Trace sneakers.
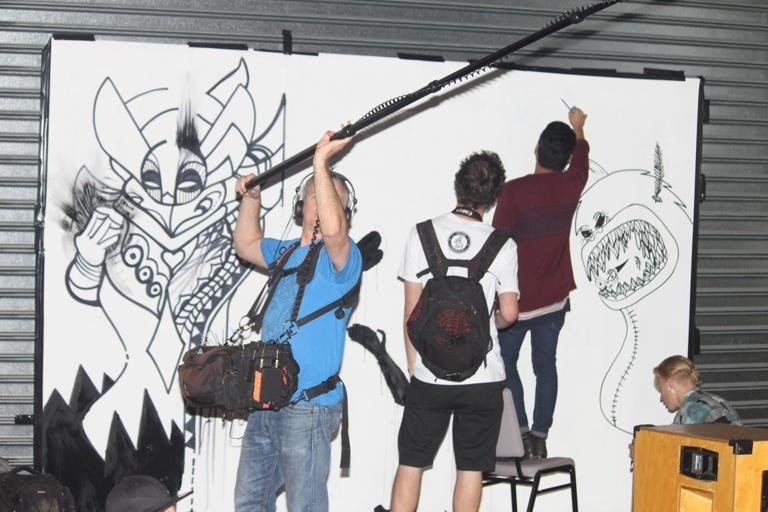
[519,432,547,461]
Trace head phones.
[292,171,357,229]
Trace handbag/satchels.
[178,340,300,421]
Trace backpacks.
[405,219,519,381]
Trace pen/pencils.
[562,99,573,111]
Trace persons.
[233,120,364,512]
[629,357,741,462]
[491,106,590,461]
[390,150,520,512]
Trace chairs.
[485,388,578,511]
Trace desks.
[633,423,767,511]
